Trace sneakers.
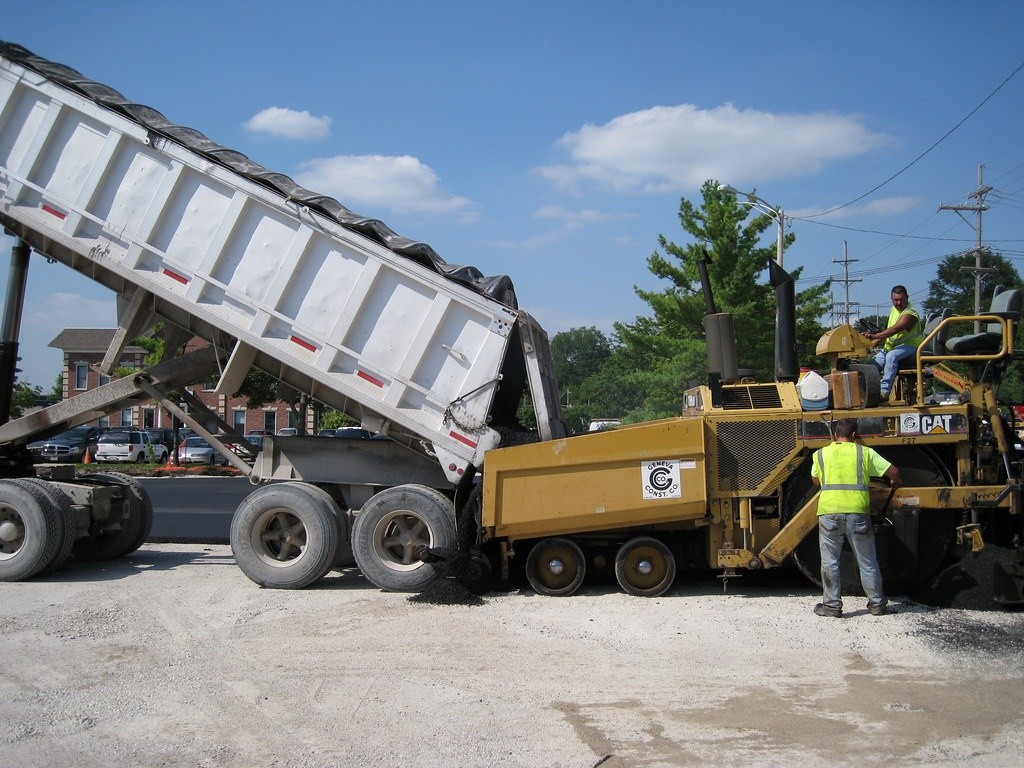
[867,602,886,615]
[814,603,842,618]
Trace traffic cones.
[83,446,92,464]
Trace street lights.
[716,184,784,382]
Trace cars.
[234,424,375,457]
[169,436,230,467]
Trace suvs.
[41,426,111,463]
[144,425,199,452]
[93,427,170,465]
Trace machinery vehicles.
[474,245,1023,609]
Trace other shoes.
[880,392,889,402]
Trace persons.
[861,285,923,403]
[810,418,902,618]
[798,360,820,382]
